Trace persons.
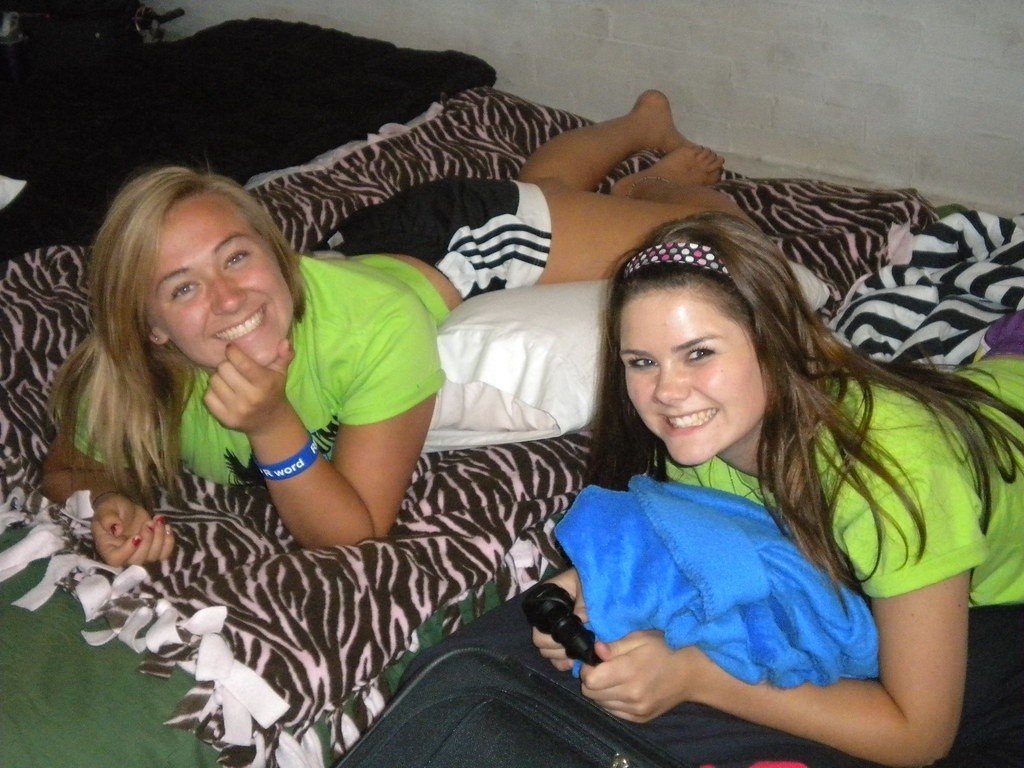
[39,85,749,569]
[529,209,1024,768]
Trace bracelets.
[251,430,320,481]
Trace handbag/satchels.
[332,647,691,768]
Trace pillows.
[420,258,832,451]
